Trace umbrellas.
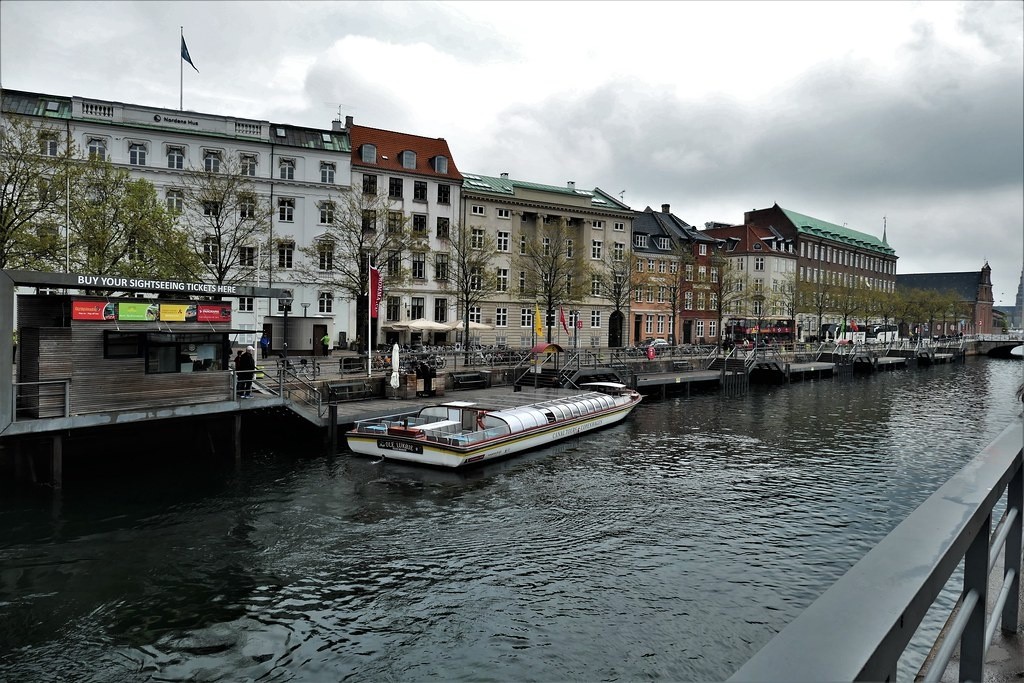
[389,342,400,398]
[381,317,495,346]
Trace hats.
[247,345,255,350]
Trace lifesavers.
[477,411,487,430]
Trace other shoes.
[240,395,246,398]
[246,394,254,398]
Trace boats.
[345,380,642,468]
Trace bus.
[820,323,899,344]
[724,317,796,351]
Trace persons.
[762,335,770,350]
[414,361,437,398]
[320,333,329,356]
[260,332,271,359]
[356,334,361,352]
[234,346,256,399]
[278,349,298,374]
[933,329,963,343]
[389,338,393,345]
[742,336,749,354]
[909,336,923,347]
[721,338,735,357]
[403,335,432,352]
[223,340,233,369]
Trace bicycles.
[372,350,448,372]
[282,360,315,382]
[471,347,523,366]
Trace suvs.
[623,338,669,356]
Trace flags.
[841,319,845,332]
[370,266,383,318]
[561,305,569,335]
[182,36,199,73]
[850,320,858,331]
[534,303,543,337]
